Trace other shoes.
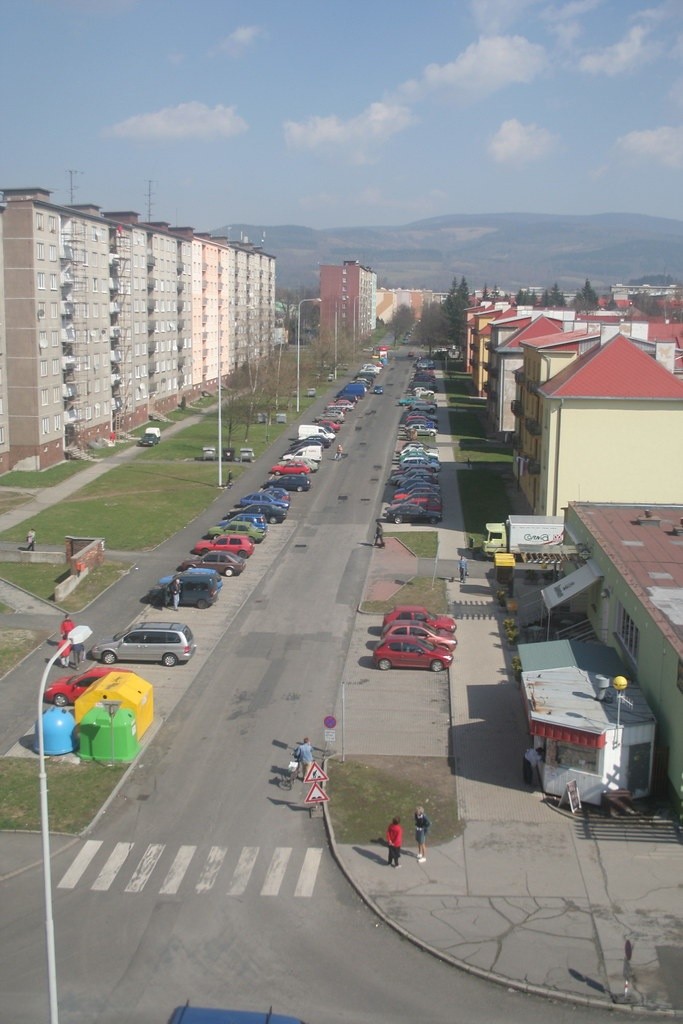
[61,664,64,668]
[64,666,68,668]
[76,666,79,670]
[417,854,422,858]
[395,864,401,870]
[418,858,426,862]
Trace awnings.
[540,561,605,641]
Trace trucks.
[297,424,336,443]
[283,445,322,463]
[482,515,564,562]
[342,384,364,390]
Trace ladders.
[116,413,120,440]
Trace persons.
[336,445,342,461]
[172,578,181,611]
[296,738,312,779]
[71,644,82,670]
[522,747,545,786]
[57,635,70,667]
[386,817,402,868]
[372,522,384,548]
[415,807,431,862]
[81,646,85,662]
[26,529,36,551]
[61,615,74,636]
[458,555,467,582]
[225,469,232,489]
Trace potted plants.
[511,656,522,682]
[503,618,520,645]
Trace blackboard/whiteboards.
[558,779,581,812]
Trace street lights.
[334,293,347,380]
[218,305,254,486]
[297,298,322,413]
[37,625,93,1024]
[353,294,367,363]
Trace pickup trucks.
[138,434,160,447]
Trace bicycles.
[289,754,300,790]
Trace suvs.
[264,475,312,492]
[148,568,223,609]
[216,514,268,532]
[91,621,196,667]
[194,534,255,559]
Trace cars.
[373,604,457,672]
[260,488,291,501]
[386,356,443,525]
[363,347,373,352]
[272,362,384,476]
[181,550,246,577]
[44,666,136,706]
[207,521,266,544]
[227,504,287,524]
[408,352,414,356]
[240,492,290,510]
[373,386,383,394]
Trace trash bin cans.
[203,447,216,461]
[240,448,253,462]
[277,414,287,424]
[306,388,316,396]
[258,413,268,423]
[223,447,235,461]
